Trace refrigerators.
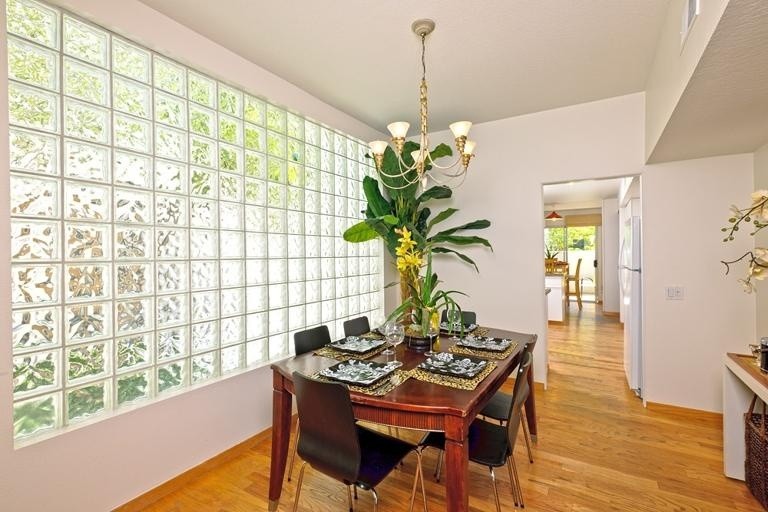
[617,196,642,402]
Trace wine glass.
[750,342,762,367]
[449,310,460,340]
[424,311,440,356]
[377,316,395,355]
[386,322,403,368]
[412,310,420,325]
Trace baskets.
[744,412,768,509]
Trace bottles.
[760,337,768,375]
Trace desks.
[722,353,768,481]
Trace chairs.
[343,315,370,337]
[408,345,532,509]
[442,310,477,327]
[286,324,331,482]
[290,369,429,512]
[566,258,582,311]
[478,334,535,466]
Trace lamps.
[545,204,563,220]
[368,17,477,190]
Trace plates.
[440,321,479,332]
[417,351,489,379]
[318,357,396,386]
[456,333,512,353]
[324,335,387,354]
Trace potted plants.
[545,244,559,268]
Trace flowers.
[721,188,768,294]
[382,226,473,343]
